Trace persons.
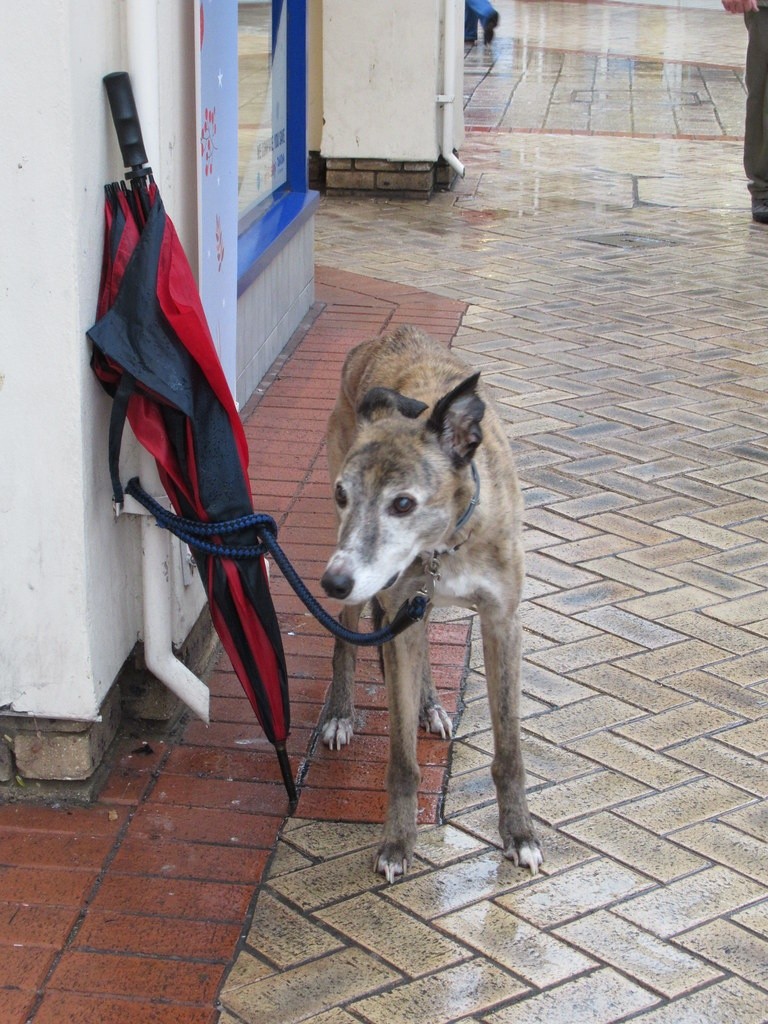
[465,0,501,45]
[721,0,768,221]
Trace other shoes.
[484,11,498,44]
[751,200,768,224]
[464,38,475,43]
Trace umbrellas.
[86,72,296,811]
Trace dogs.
[314,321,548,887]
